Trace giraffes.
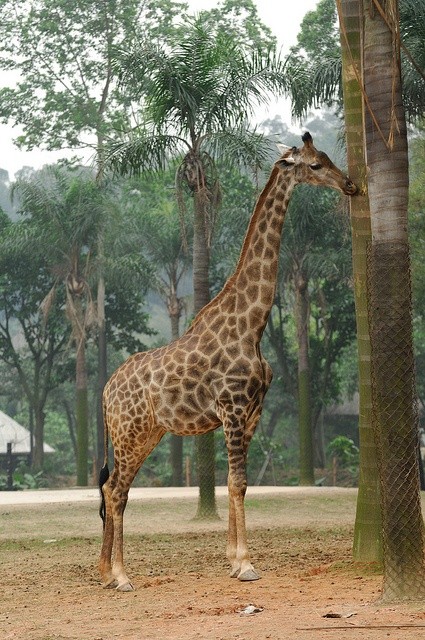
[98,130,358,592]
